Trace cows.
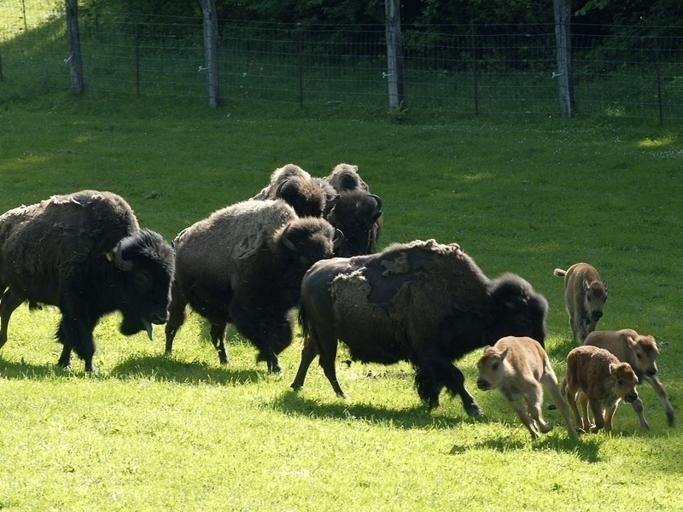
[549,260,611,341]
[0,185,179,375]
[251,160,388,266]
[166,195,346,377]
[475,334,584,450]
[289,236,552,422]
[585,325,679,437]
[554,342,646,434]
